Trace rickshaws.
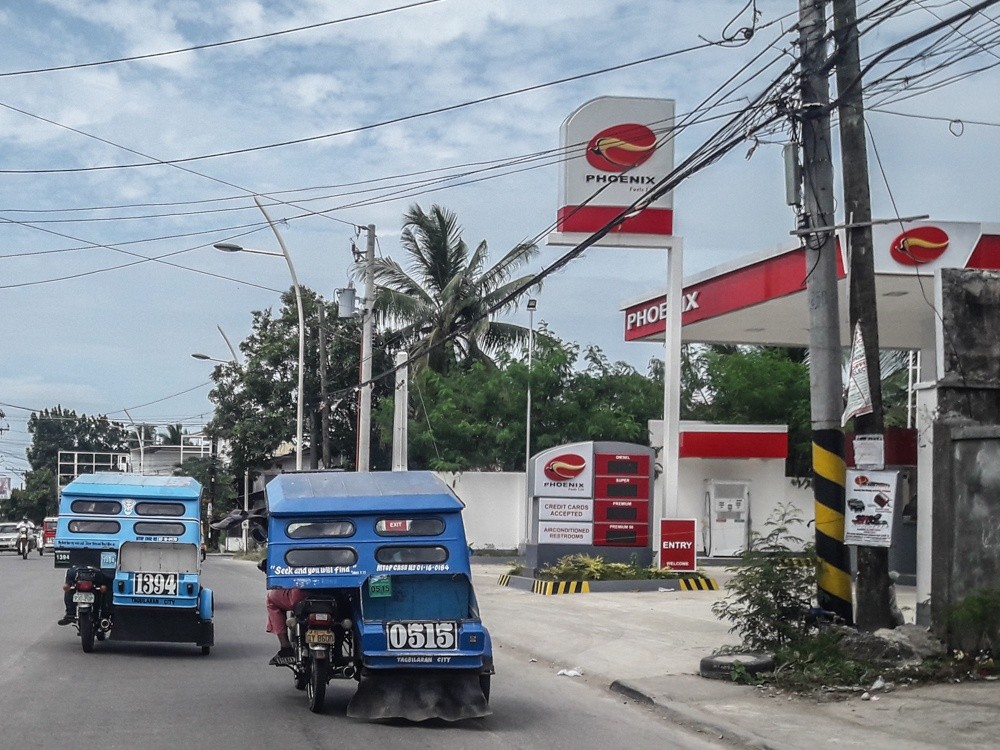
[251,467,496,724]
[53,471,216,656]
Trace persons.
[257,523,335,658]
[58,502,119,625]
[16,516,36,551]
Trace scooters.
[13,524,43,559]
[33,517,58,548]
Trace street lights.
[213,195,306,471]
[191,323,251,555]
[518,297,537,556]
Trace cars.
[0,522,21,550]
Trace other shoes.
[58,614,77,626]
[268,647,296,666]
